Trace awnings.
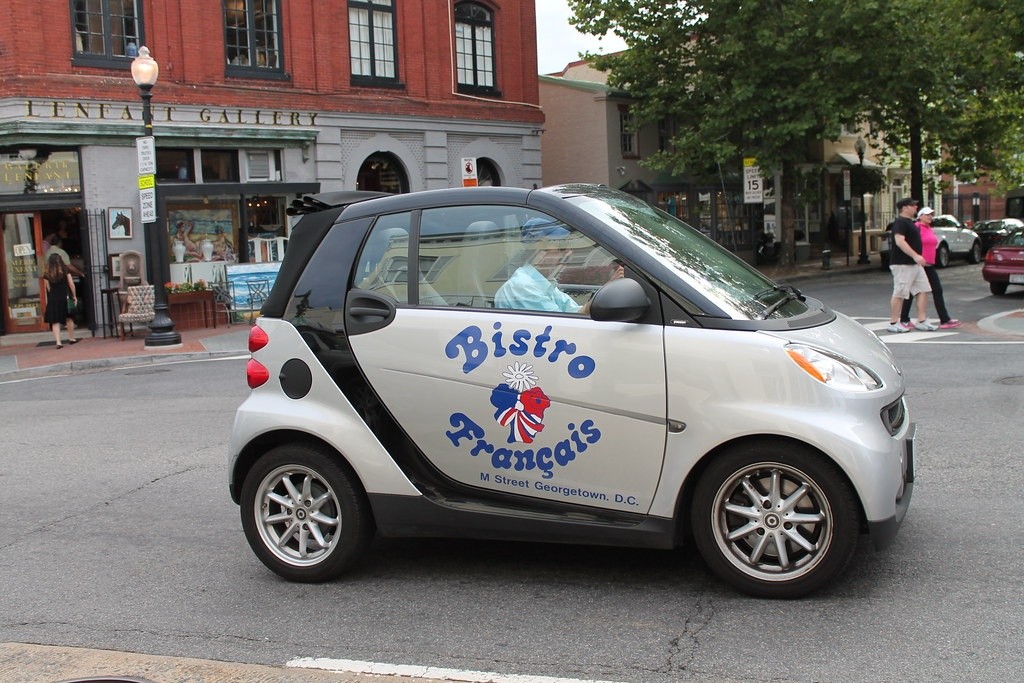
[836,153,886,170]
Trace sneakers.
[916,318,938,331]
[888,321,910,333]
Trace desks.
[167,290,217,329]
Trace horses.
[112,211,130,236]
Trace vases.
[172,240,185,263]
[201,239,213,262]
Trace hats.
[897,197,919,209]
[916,207,935,221]
[521,221,571,250]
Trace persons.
[494,216,624,315]
[42,227,56,253]
[44,235,85,328]
[900,207,962,331]
[44,253,79,349]
[888,198,939,331]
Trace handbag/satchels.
[67,299,82,313]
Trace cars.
[980,226,1024,296]
[224,182,918,600]
[970,215,1024,257]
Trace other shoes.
[57,344,64,349]
[940,318,961,329]
[70,338,77,344]
[901,319,916,329]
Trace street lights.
[852,136,872,265]
[129,45,184,346]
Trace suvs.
[877,213,984,272]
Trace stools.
[101,288,134,338]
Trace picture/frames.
[165,201,239,264]
[109,253,121,280]
[108,206,133,240]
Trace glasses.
[542,239,573,251]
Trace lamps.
[617,165,626,176]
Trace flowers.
[164,279,207,294]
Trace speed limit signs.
[741,156,764,205]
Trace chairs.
[245,280,271,325]
[119,285,155,342]
[368,227,447,308]
[248,236,289,263]
[211,281,237,327]
[457,220,511,309]
